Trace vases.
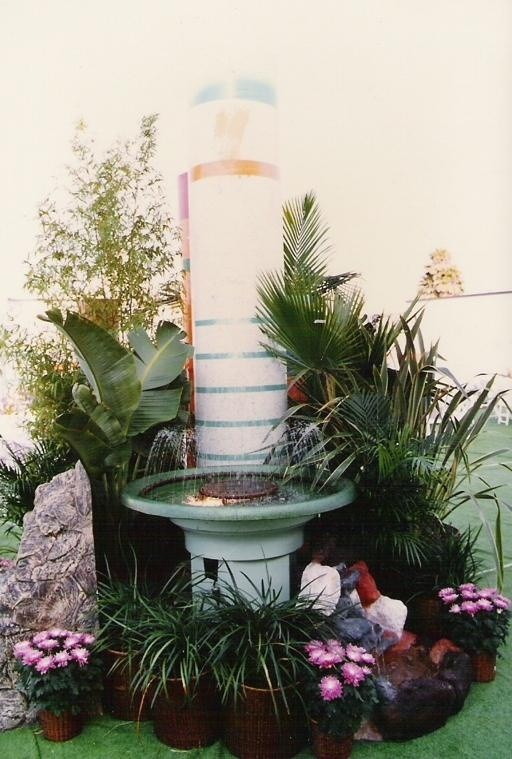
[463,655,499,684]
[39,705,80,740]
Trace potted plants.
[83,543,362,759]
[270,288,509,633]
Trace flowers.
[435,581,509,662]
[12,625,98,722]
[304,636,383,740]
